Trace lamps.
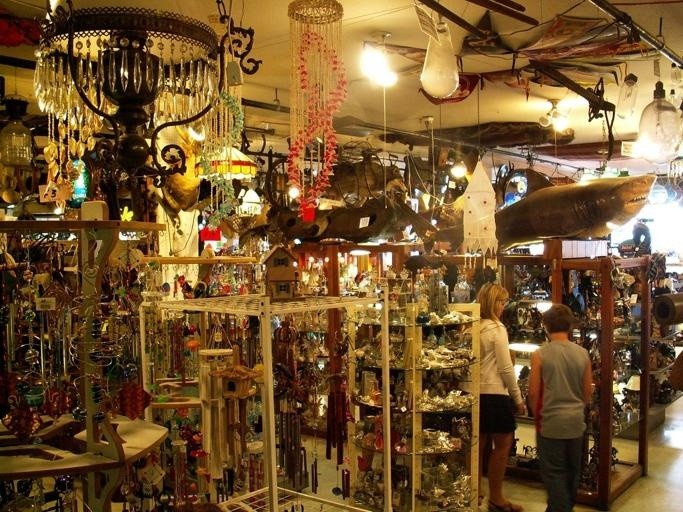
[33,0,224,225]
[195,143,258,185]
[450,142,467,181]
[634,41,682,161]
[535,92,565,129]
[0,61,33,170]
[237,185,262,220]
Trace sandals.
[487,499,523,512]
[477,495,484,506]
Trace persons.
[527,303,592,512]
[458,282,525,512]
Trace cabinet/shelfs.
[0,210,681,511]
